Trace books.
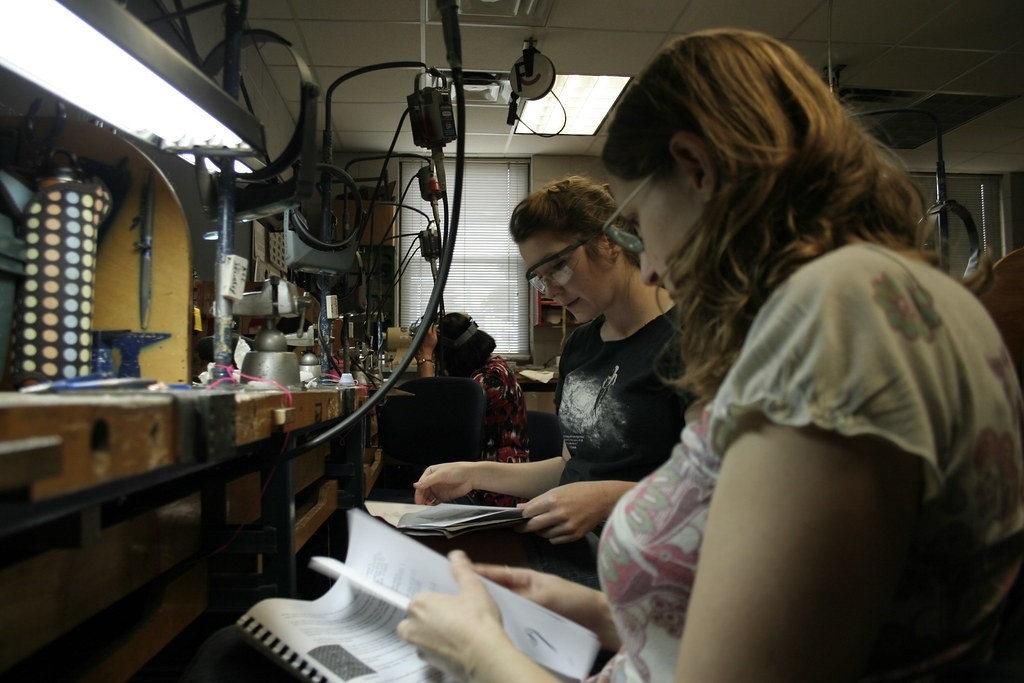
[236,501,600,683]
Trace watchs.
[417,359,435,364]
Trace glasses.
[603,166,661,252]
[525,235,595,295]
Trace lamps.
[0,0,267,174]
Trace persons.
[411,313,530,508]
[410,175,704,589]
[396,27,1023,683]
